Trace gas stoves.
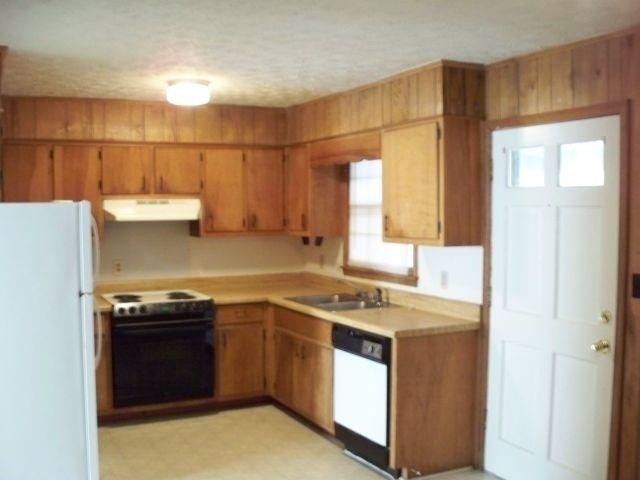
[99,287,215,319]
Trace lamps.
[166,78,210,106]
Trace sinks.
[317,302,400,313]
[283,293,360,305]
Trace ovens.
[108,311,220,414]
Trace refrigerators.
[1,199,103,480]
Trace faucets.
[354,288,381,305]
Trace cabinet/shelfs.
[190,146,287,237]
[284,142,349,238]
[212,302,267,409]
[102,142,203,194]
[381,115,484,247]
[0,140,104,242]
[266,303,336,436]
